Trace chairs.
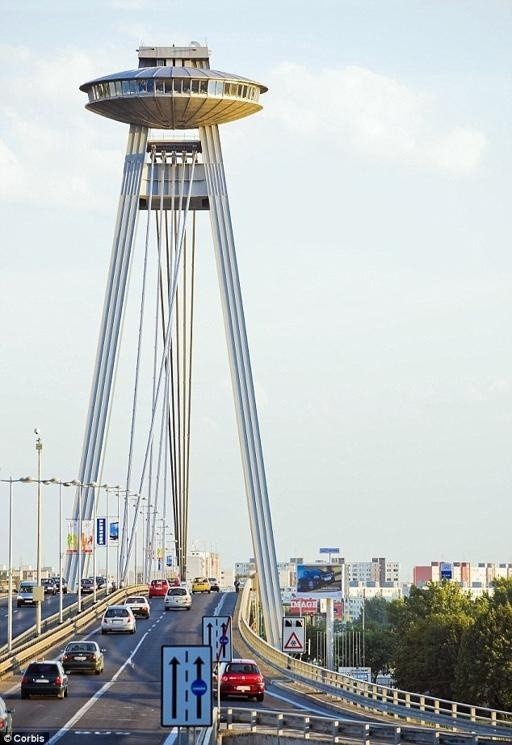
[244,666,250,673]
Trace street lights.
[0,475,187,651]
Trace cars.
[164,587,193,610]
[298,569,335,590]
[191,576,220,594]
[212,658,264,701]
[0,640,106,745]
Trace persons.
[234,579,241,593]
